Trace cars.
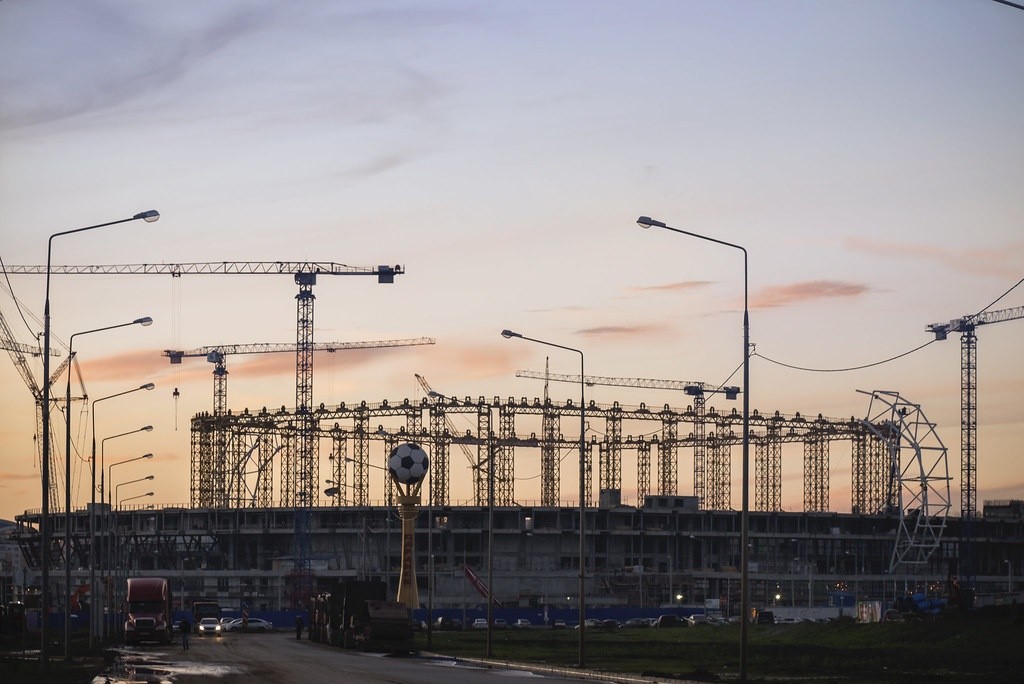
[512,619,532,630]
[198,618,223,638]
[494,618,508,631]
[554,618,567,629]
[577,610,829,627]
[219,616,273,632]
[472,618,488,630]
[415,616,462,632]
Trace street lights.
[501,328,588,667]
[326,479,366,585]
[638,214,750,683]
[41,208,159,678]
[66,316,153,666]
[428,391,493,658]
[108,453,153,653]
[114,475,155,640]
[100,426,154,646]
[91,383,157,660]
[345,456,393,602]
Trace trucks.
[192,599,222,631]
[121,574,175,647]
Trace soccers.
[388,444,429,483]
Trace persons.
[296,615,304,640]
[179,615,191,651]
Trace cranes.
[162,337,439,624]
[0,260,410,407]
[512,366,744,508]
[0,313,89,510]
[925,305,1024,521]
[414,371,481,509]
